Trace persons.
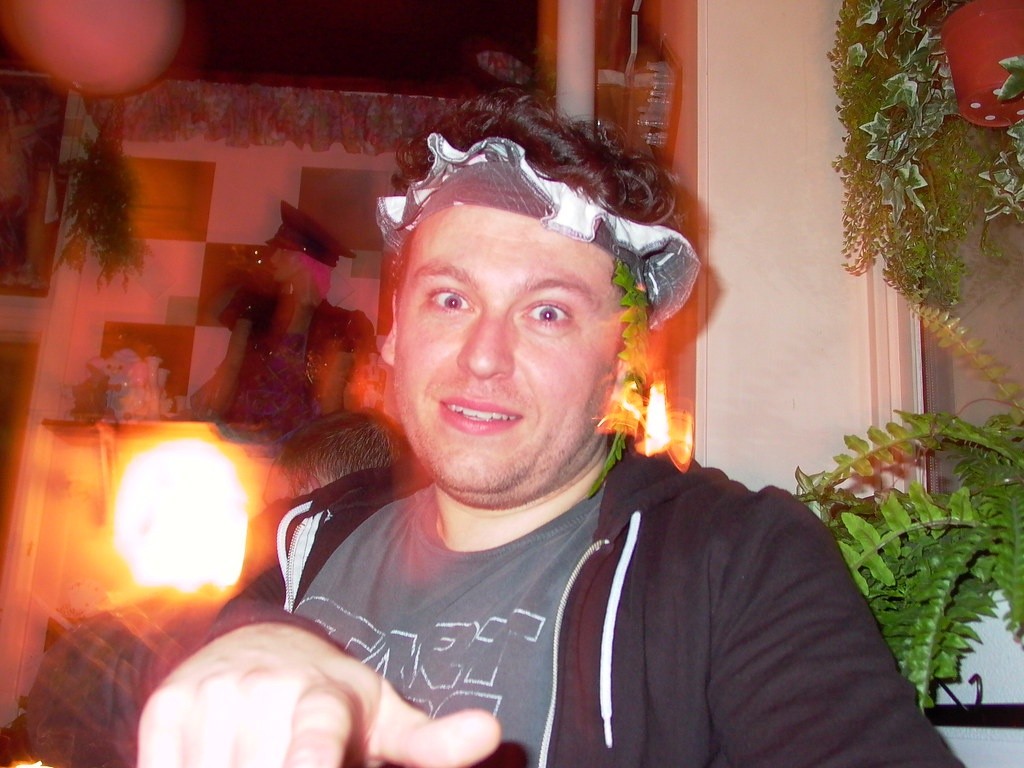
[260,407,403,506]
[30,82,961,768]
